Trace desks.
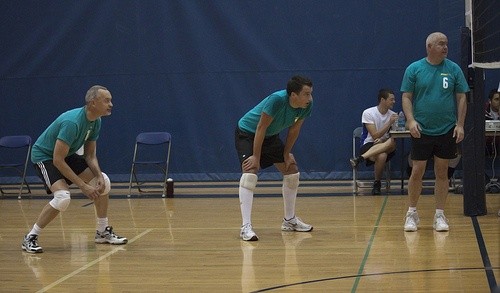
[388,128,413,187]
[485,132,500,160]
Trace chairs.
[0,136,33,198]
[127,131,173,197]
[352,127,391,195]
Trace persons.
[20,85,128,253]
[235,77,313,241]
[349,90,398,195]
[485,90,500,193]
[401,32,469,232]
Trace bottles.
[393,109,406,131]
[166,177,175,198]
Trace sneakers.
[281,216,313,231]
[372,181,381,194]
[21,233,43,253]
[350,158,358,167]
[433,213,448,231]
[240,223,258,241]
[95,226,128,244]
[404,212,418,231]
